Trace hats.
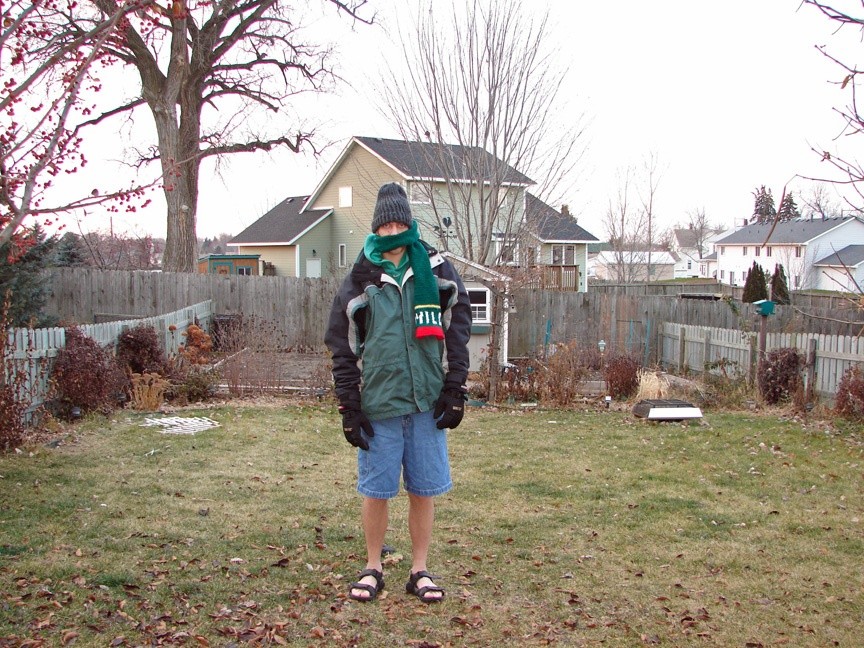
[371,182,412,233]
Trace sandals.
[406,570,445,602]
[349,569,384,601]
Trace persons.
[325,183,472,599]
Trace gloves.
[433,386,468,430]
[337,400,375,451]
